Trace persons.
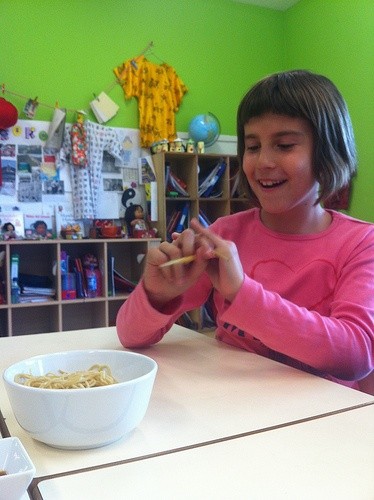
[3,222,20,240]
[125,203,158,238]
[116,69,374,391]
[33,221,52,240]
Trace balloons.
[0,97,18,129]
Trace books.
[175,301,216,331]
[230,164,247,198]
[11,252,57,304]
[167,202,191,243]
[165,164,191,197]
[71,257,87,300]
[199,161,226,198]
[199,207,212,229]
[107,249,139,298]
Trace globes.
[189,111,221,147]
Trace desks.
[31,401,374,500]
[0,322,374,494]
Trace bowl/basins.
[91,226,122,239]
[0,436,37,499]
[3,349,158,451]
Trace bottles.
[150,137,205,153]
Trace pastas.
[14,364,122,390]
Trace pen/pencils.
[159,250,216,268]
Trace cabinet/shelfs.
[150,151,251,337]
[0,236,164,337]
